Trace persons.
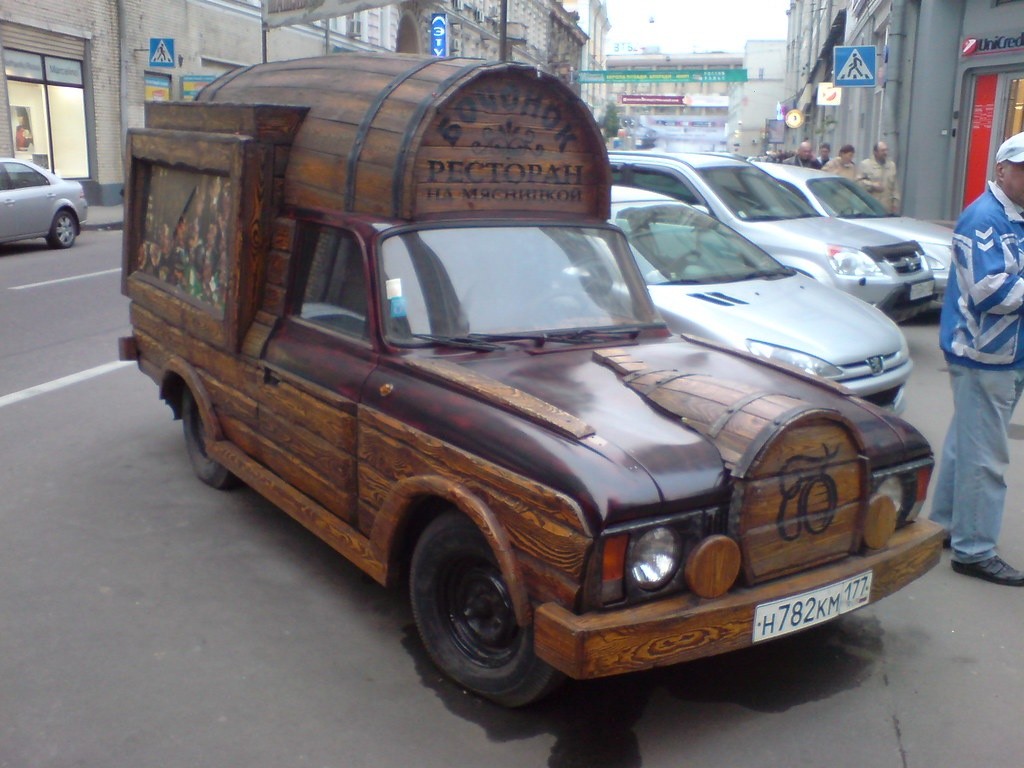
[755,141,901,216]
[929,131,1024,588]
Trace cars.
[743,156,956,312]
[0,157,90,250]
[555,182,914,416]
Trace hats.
[996,131,1024,164]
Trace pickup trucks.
[113,48,946,715]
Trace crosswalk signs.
[832,45,877,89]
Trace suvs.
[606,143,940,323]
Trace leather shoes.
[943,535,951,549]
[951,555,1024,585]
